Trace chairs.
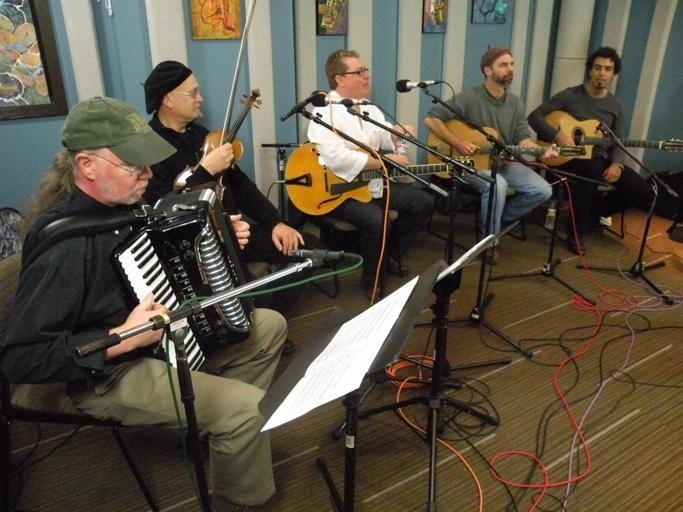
[0,206,157,512]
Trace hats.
[59,95,178,167]
[480,47,512,72]
[144,60,193,114]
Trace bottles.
[395,138,408,158]
[544,199,556,230]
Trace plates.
[0,0,44,103]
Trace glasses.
[159,88,200,98]
[332,67,369,77]
[75,148,145,177]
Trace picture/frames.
[0,1,71,123]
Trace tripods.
[414,161,533,358]
[489,179,597,305]
[358,294,498,512]
[578,184,673,304]
[399,181,513,434]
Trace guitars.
[285,140,477,216]
[537,110,683,167]
[426,120,587,178]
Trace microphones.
[396,79,441,92]
[291,249,341,261]
[311,90,373,107]
[280,94,316,122]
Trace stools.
[533,174,626,254]
[296,207,405,301]
[428,172,527,259]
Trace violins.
[173,88,262,201]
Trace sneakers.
[565,219,585,256]
[208,492,257,512]
[383,257,409,278]
[482,246,501,265]
[283,337,296,356]
[360,275,386,292]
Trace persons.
[304,47,439,304]
[524,46,655,256]
[143,59,326,359]
[0,93,292,510]
[423,46,561,268]
